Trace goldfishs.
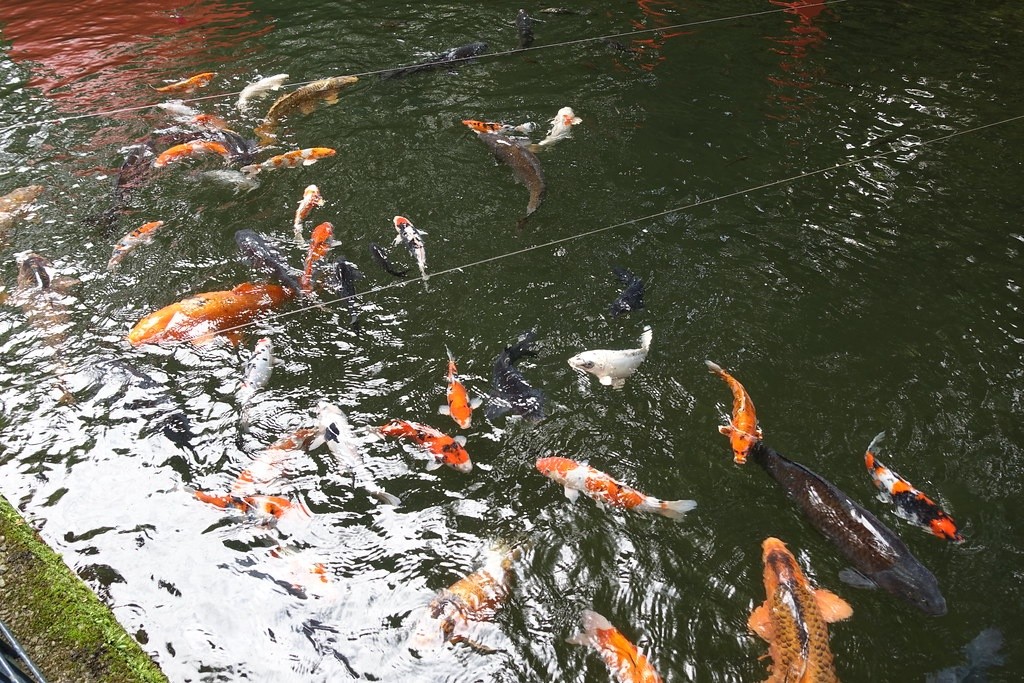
[0,6,1008,683]
